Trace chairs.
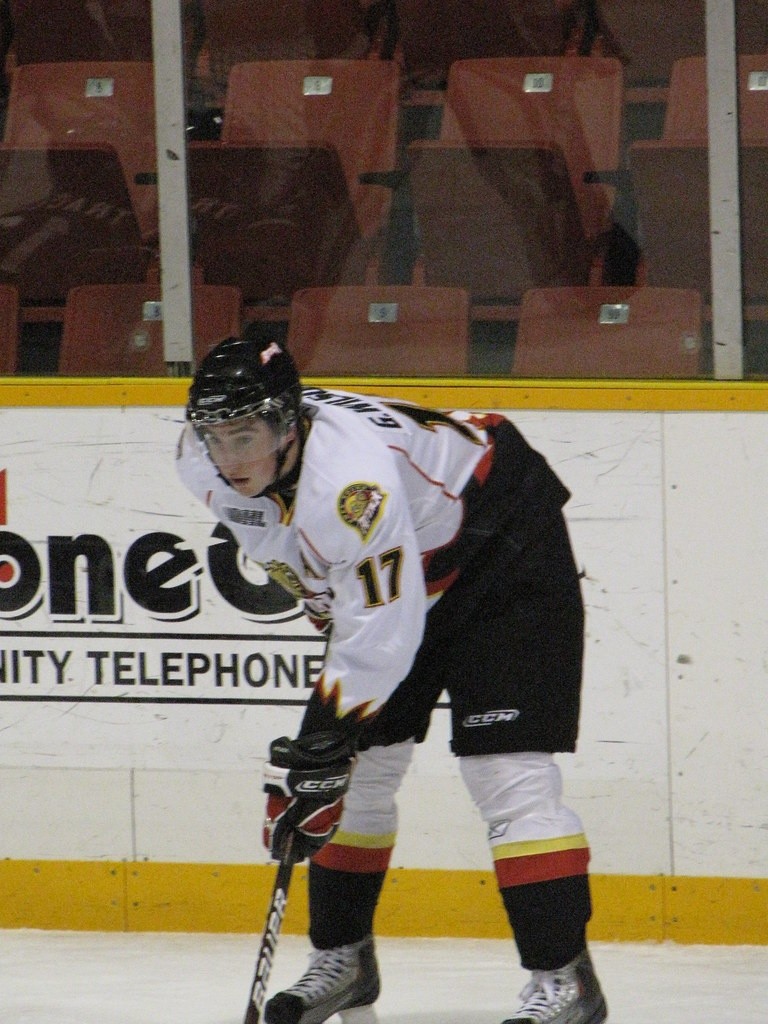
[406,58,626,315]
[510,288,703,378]
[188,57,404,289]
[285,287,471,378]
[61,284,242,375]
[630,53,768,302]
[0,63,158,300]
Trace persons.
[175,334,607,1024]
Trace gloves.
[263,732,357,864]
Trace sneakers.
[265,930,380,1024]
[503,947,608,1024]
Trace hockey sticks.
[242,624,334,1024]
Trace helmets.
[186,337,302,428]
